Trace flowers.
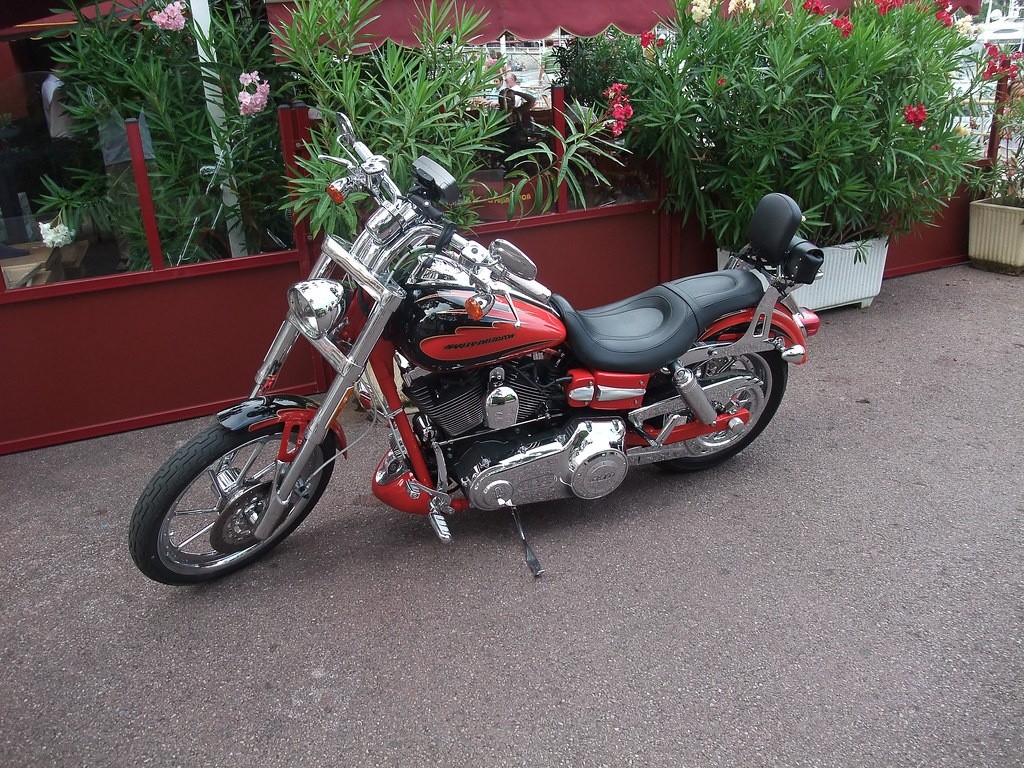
[594,0,1024,265]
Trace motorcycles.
[126,108,824,588]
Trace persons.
[41,60,88,190]
[483,39,563,108]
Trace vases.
[969,197,1024,276]
[716,235,889,312]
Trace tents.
[263,0,680,64]
[0,0,192,39]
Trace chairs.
[491,126,554,171]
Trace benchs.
[44,237,91,281]
